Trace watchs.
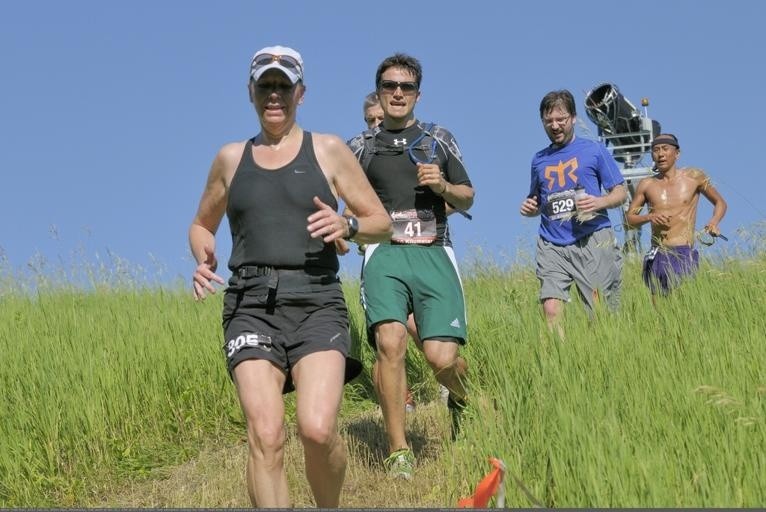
[341,213,359,242]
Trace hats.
[251,46,303,85]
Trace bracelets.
[435,178,447,198]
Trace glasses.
[383,80,419,95]
[699,231,715,247]
[250,53,302,74]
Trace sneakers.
[405,385,416,412]
[386,448,418,483]
[447,393,467,442]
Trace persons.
[623,133,729,310]
[187,45,396,511]
[341,90,472,419]
[334,51,477,484]
[520,89,628,343]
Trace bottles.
[574,184,593,221]
